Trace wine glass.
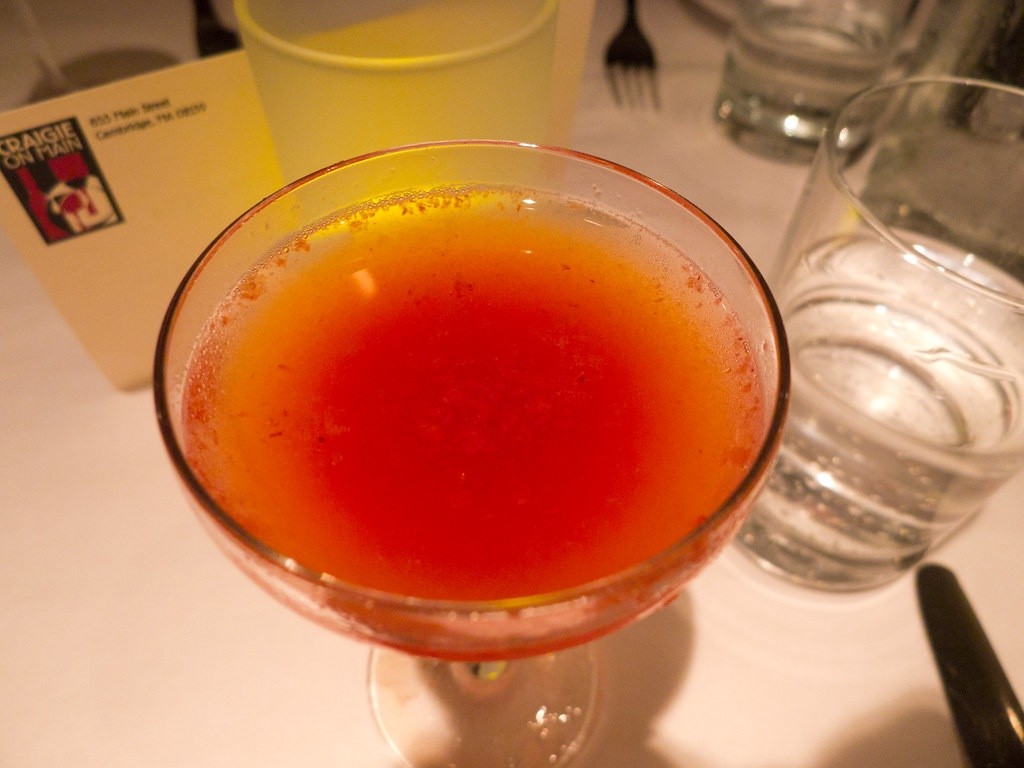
[148,136,791,767]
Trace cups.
[743,72,1022,596]
[718,1,916,164]
[235,0,556,186]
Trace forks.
[606,2,660,107]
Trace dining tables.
[0,0,1020,764]
